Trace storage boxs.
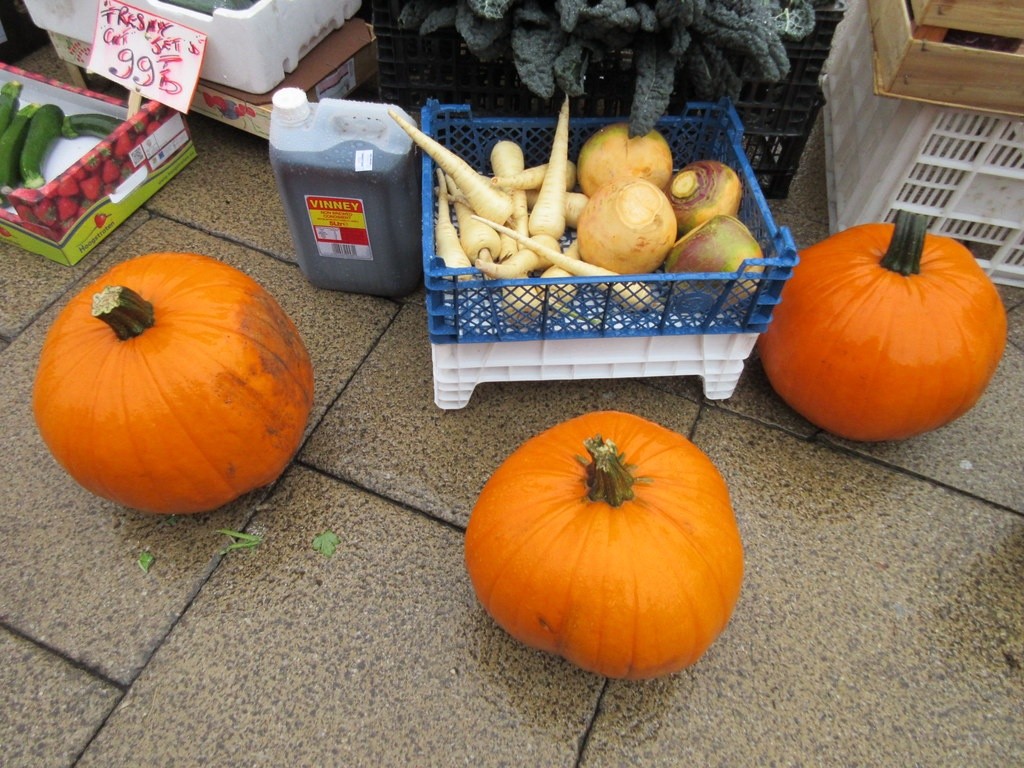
[370,1,846,137]
[822,1,1024,289]
[419,91,800,409]
[424,90,827,199]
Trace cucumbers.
[0,80,128,208]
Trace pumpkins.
[465,410,745,680]
[35,253,315,515]
[757,209,1007,440]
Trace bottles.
[268,85,424,299]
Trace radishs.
[577,123,765,309]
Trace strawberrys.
[9,102,192,242]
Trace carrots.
[389,89,652,336]
